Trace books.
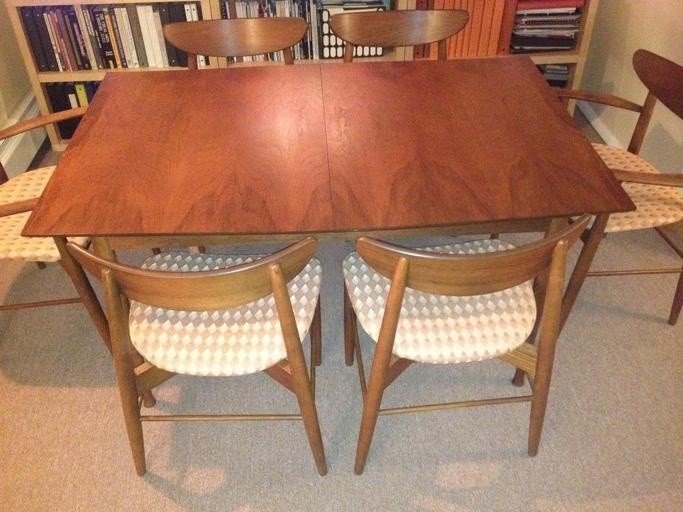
[509,0,585,90]
[40,81,103,139]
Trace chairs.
[65,235,328,476]
[1,10,683,407]
[343,213,593,475]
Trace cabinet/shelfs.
[4,0,601,150]
